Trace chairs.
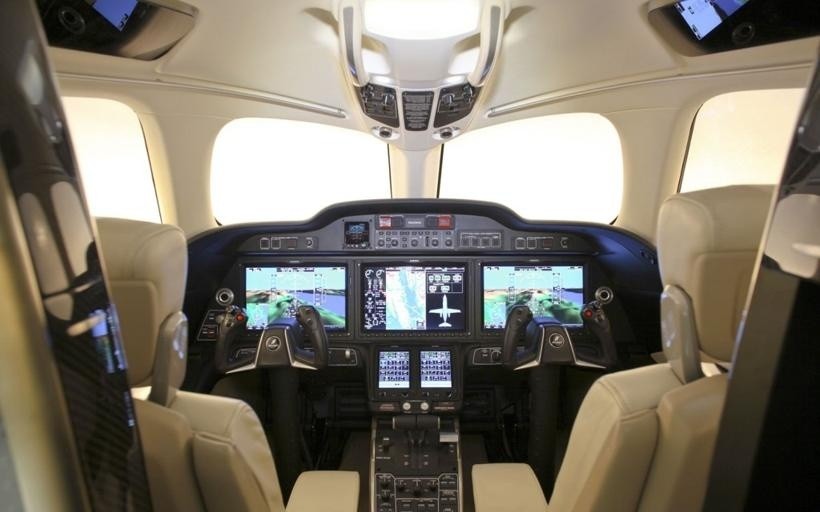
[471,186,782,512]
[94,217,361,512]
